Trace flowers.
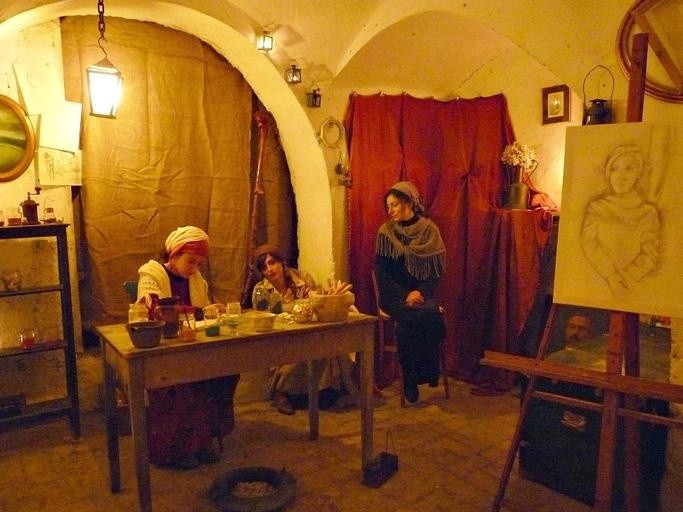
[501,141,537,177]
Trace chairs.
[370,269,449,407]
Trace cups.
[20,329,36,350]
[226,301,241,314]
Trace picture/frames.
[542,84,570,124]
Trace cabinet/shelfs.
[0,223,83,444]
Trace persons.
[375,180,448,404]
[136,225,241,469]
[251,244,359,415]
[562,314,593,351]
[582,147,661,304]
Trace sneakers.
[405,381,419,403]
[174,455,199,468]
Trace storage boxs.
[518,374,670,511]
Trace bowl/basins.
[248,313,277,332]
[124,322,166,347]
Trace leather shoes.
[274,394,294,414]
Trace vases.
[504,166,528,209]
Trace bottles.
[128,303,149,320]
[44,208,55,224]
[6,207,22,225]
[154,298,220,342]
[291,299,313,324]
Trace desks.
[94,297,379,511]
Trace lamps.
[86,0,124,119]
[256,30,274,51]
[306,83,321,108]
[285,58,301,84]
